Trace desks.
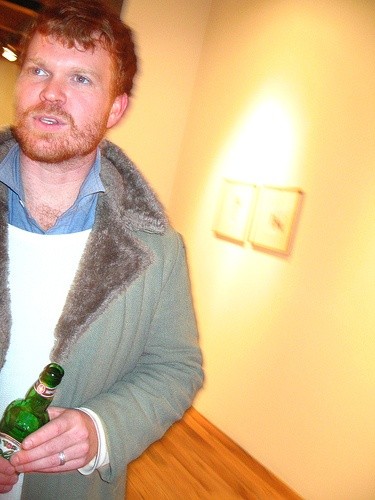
[125,407,305,500]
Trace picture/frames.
[250,184,305,258]
[213,179,255,247]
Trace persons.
[0,0,205,500]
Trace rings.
[58,450,66,466]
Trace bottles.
[0,363,65,460]
